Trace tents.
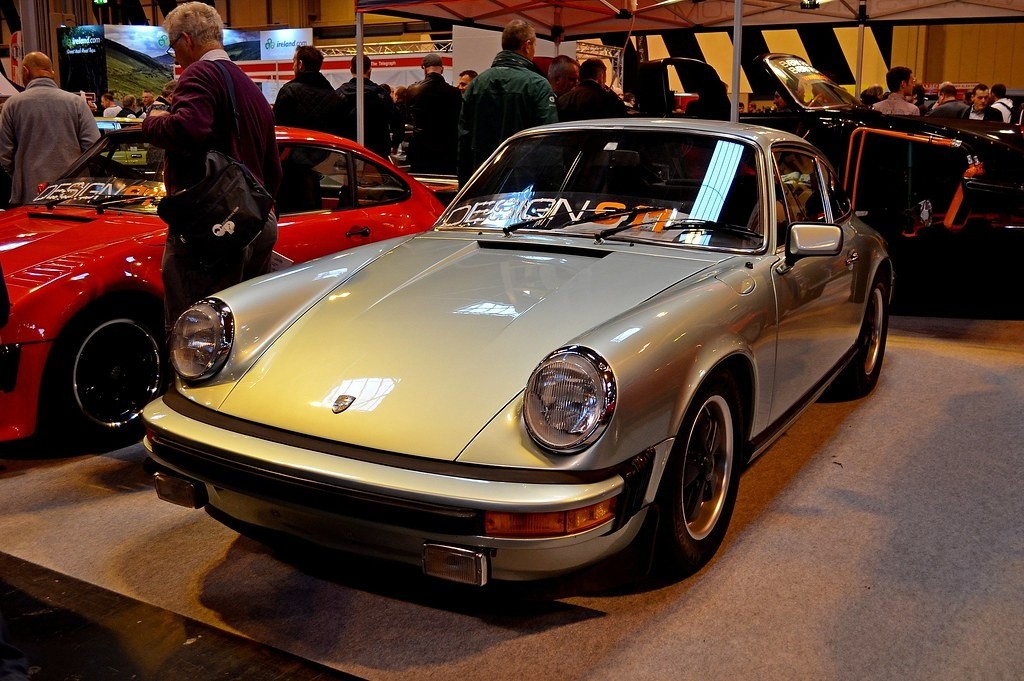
[352,0,1024,172]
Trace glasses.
[166,36,183,58]
[912,78,917,84]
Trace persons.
[100,79,177,118]
[269,103,276,110]
[335,55,405,159]
[796,171,838,223]
[0,51,101,205]
[379,20,1024,207]
[272,45,341,136]
[846,230,871,303]
[142,1,282,341]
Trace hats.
[420,53,442,68]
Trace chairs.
[569,149,651,218]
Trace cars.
[750,54,1024,320]
[137,118,894,587]
[0,118,459,453]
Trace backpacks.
[997,100,1024,126]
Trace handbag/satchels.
[157,162,275,259]
